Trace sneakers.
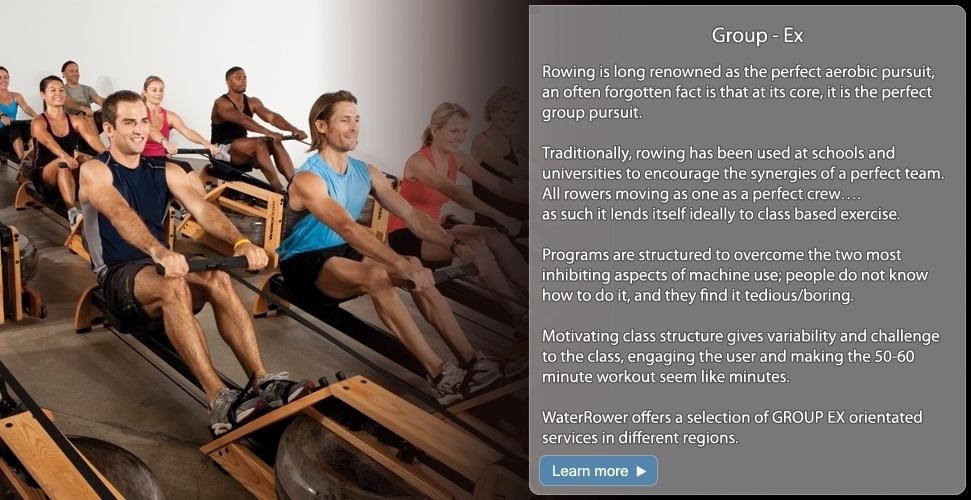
[206,387,268,440]
[67,207,83,231]
[254,367,316,410]
[427,350,527,407]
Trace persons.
[0,60,529,438]
